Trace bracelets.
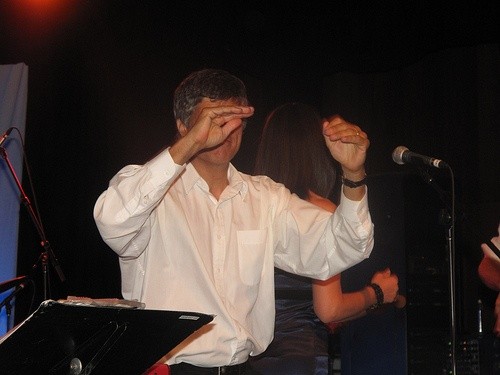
[341,176,369,188]
[368,282,384,304]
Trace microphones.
[0,126,13,146]
[392,146,446,168]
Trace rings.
[354,128,360,136]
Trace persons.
[92,68,375,375]
[247,101,400,375]
[477,222,500,375]
